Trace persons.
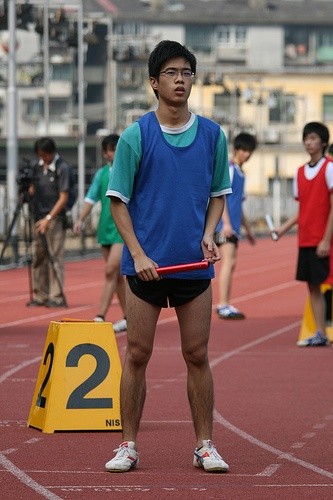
[105,40,233,473]
[73,133,142,332]
[25,136,70,307]
[265,122,333,346]
[214,131,259,320]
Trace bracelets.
[46,214,51,221]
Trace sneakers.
[297,333,328,346]
[192,439,230,472]
[216,306,245,320]
[103,441,141,472]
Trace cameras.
[14,166,37,184]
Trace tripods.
[0,184,68,308]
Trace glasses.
[160,67,194,78]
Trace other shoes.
[27,300,43,307]
[94,316,105,322]
[113,319,128,332]
[44,300,66,307]
